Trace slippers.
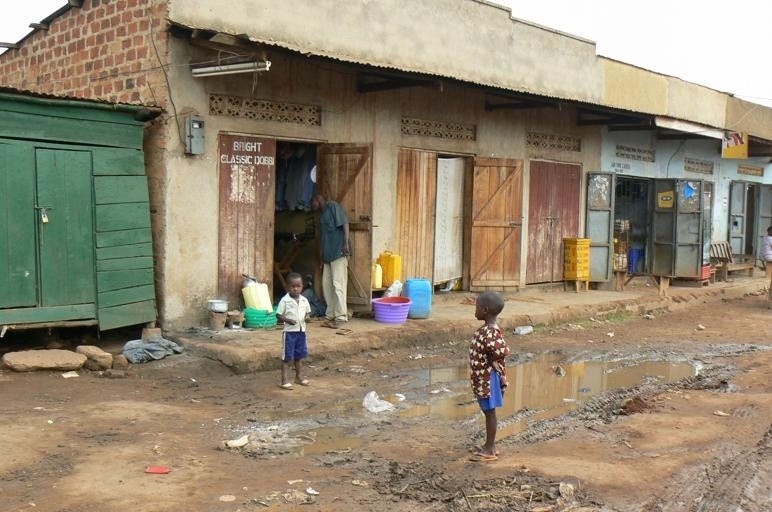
[470,452,498,462]
[280,383,294,390]
[298,379,311,386]
[469,445,500,455]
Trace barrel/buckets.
[376,249,403,288]
[401,277,433,319]
[370,263,383,290]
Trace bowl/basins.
[207,300,230,313]
[372,296,412,324]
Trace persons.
[276,273,311,389]
[759,227,772,262]
[311,195,352,328]
[469,290,511,461]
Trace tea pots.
[242,273,257,288]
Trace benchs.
[612,268,677,297]
[711,241,755,281]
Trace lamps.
[191,61,271,78]
